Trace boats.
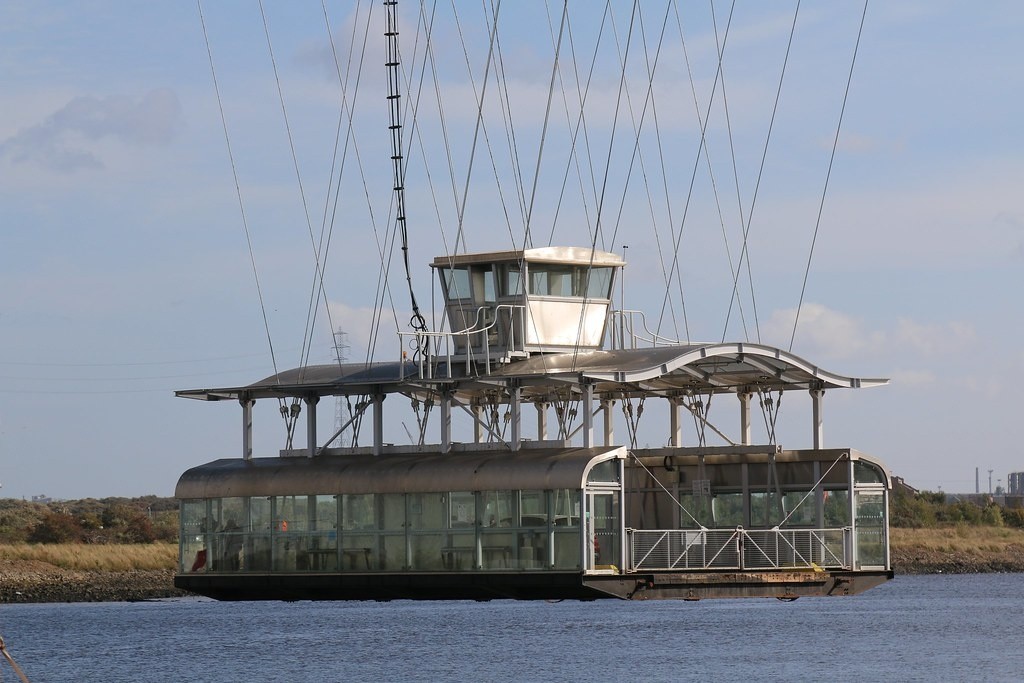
[175,1,896,600]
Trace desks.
[440,546,511,569]
[306,548,371,569]
